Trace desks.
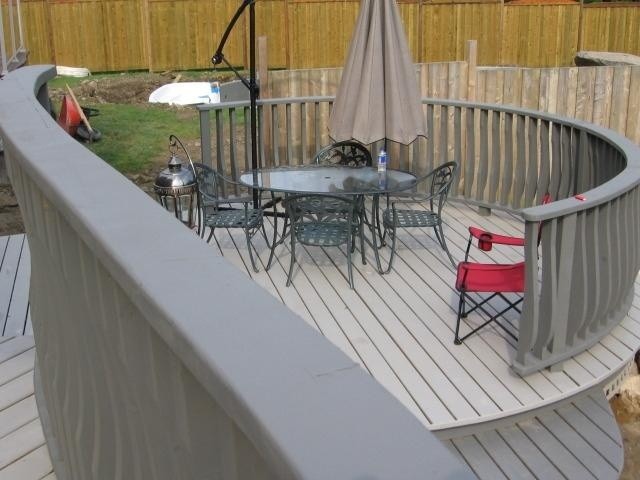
[239,164,417,273]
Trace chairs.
[189,163,272,273]
[290,142,373,253]
[281,193,365,287]
[378,161,456,274]
[454,190,554,345]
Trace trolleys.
[57,82,101,144]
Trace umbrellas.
[209,0,431,209]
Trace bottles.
[377,147,387,173]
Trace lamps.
[152,133,202,234]
